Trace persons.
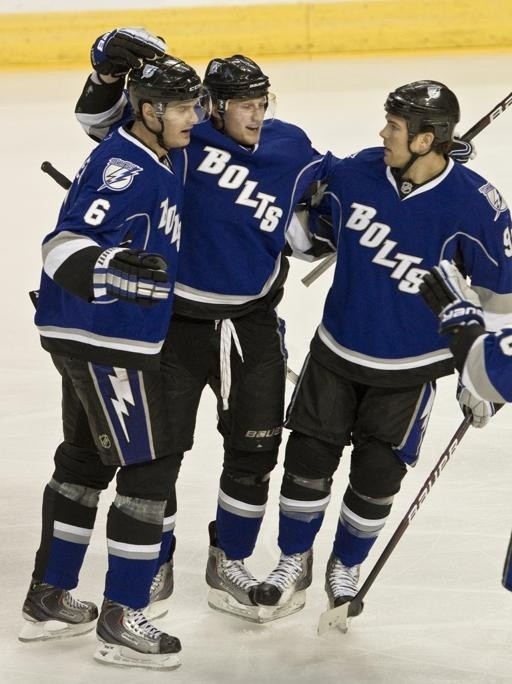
[76,27,476,607]
[415,258,511,589]
[251,78,511,617]
[20,56,213,652]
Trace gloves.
[86,25,169,79]
[417,258,487,334]
[456,375,499,429]
[445,136,479,165]
[92,246,172,307]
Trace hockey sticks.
[42,162,300,388]
[317,411,472,635]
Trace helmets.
[205,55,276,127]
[120,55,212,125]
[385,79,461,133]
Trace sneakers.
[325,550,366,621]
[205,518,311,623]
[93,534,187,669]
[15,575,100,641]
